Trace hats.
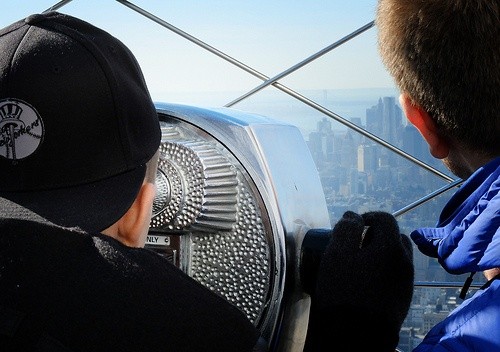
[0,11,162,235]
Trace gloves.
[304,210,414,352]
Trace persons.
[376,0,499,352]
[1,12,414,351]
